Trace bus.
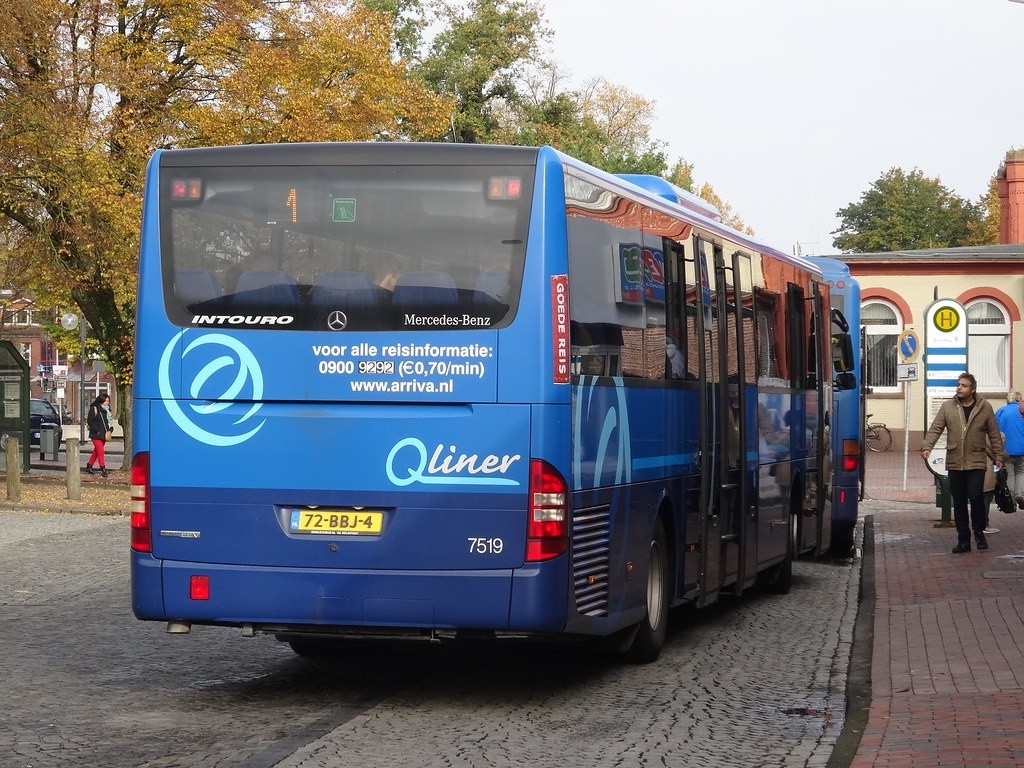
[127,136,870,667]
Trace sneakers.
[974,532,988,550]
[952,541,972,553]
[983,526,1001,534]
[1015,496,1024,510]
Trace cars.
[0,399,63,452]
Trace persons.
[222,250,280,304]
[920,373,1024,553]
[85,394,113,477]
[353,252,401,321]
[447,264,478,307]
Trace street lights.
[60,297,88,443]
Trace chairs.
[232,270,299,306]
[391,270,460,307]
[311,271,377,311]
[471,266,509,307]
[174,268,222,304]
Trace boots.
[99,465,107,477]
[85,463,95,474]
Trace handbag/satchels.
[86,406,98,431]
[994,469,1016,514]
[105,431,112,442]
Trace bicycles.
[865,412,895,454]
